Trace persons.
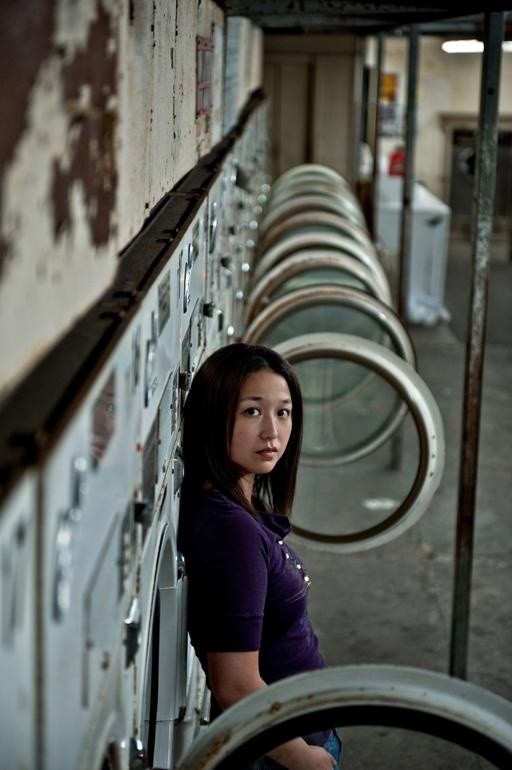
[175,343,345,769]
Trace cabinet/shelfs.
[440,112,512,235]
